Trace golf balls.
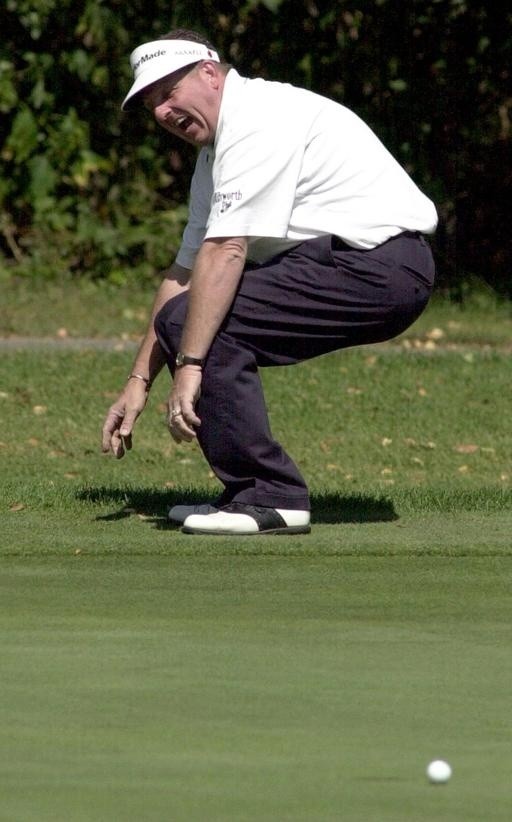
[427,760,453,783]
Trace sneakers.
[168,501,311,536]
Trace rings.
[170,408,182,415]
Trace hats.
[120,39,221,113]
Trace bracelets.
[174,351,204,368]
[126,372,152,387]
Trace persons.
[98,28,441,534]
